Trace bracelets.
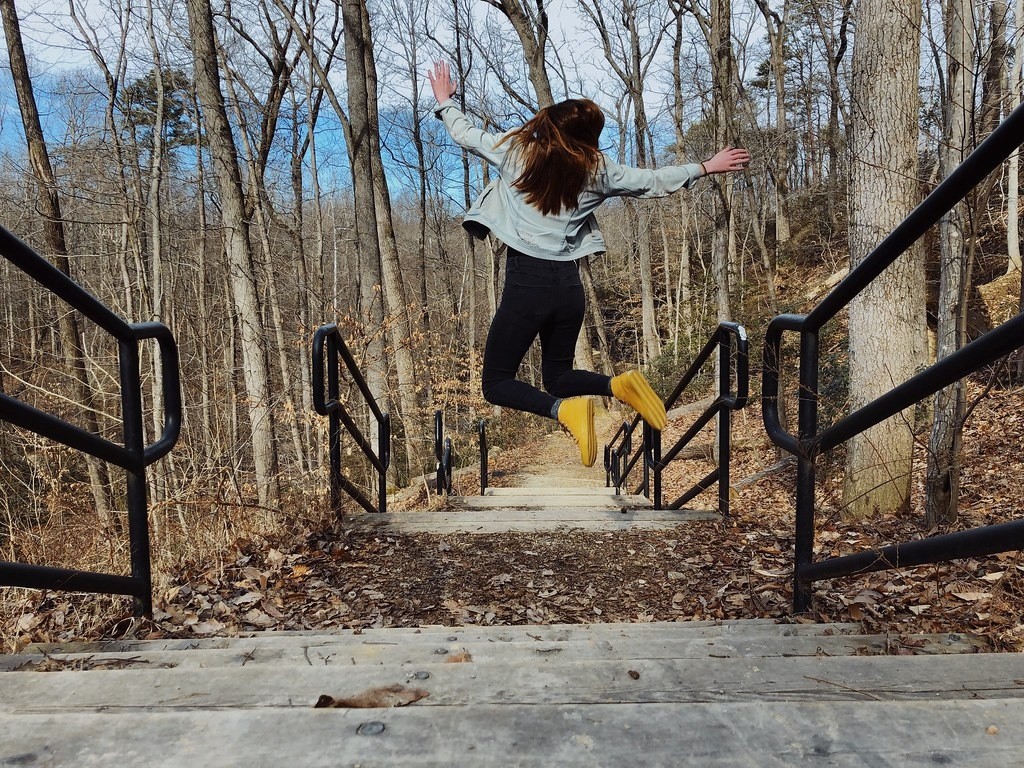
[701,163,707,174]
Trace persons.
[428,59,749,467]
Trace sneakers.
[610,368,667,431]
[557,397,598,468]
